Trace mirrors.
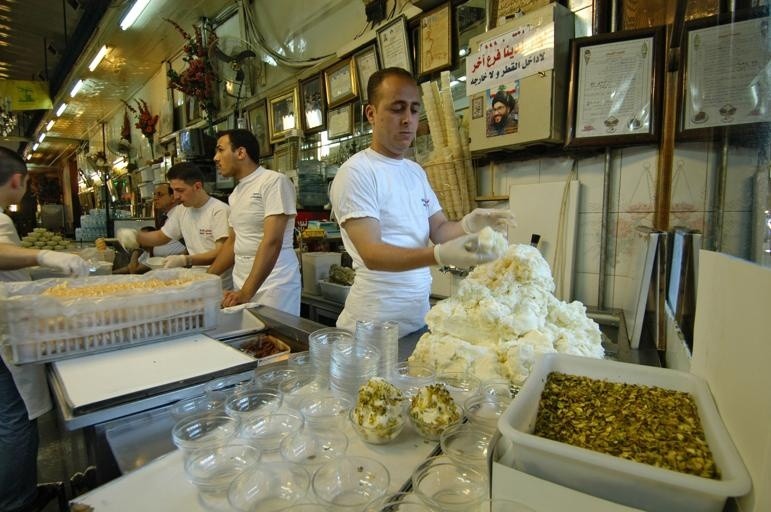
[671,0,769,354]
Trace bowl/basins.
[170,366,389,509]
[289,318,400,397]
[21,228,74,250]
[350,359,513,511]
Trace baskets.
[0,265,223,366]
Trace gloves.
[434,227,509,266]
[459,207,517,236]
[36,247,91,280]
[162,252,186,270]
[116,227,140,251]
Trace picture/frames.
[245,4,462,164]
[560,23,668,151]
[675,6,769,143]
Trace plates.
[147,256,164,265]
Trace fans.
[208,31,264,97]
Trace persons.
[0,146,90,512]
[111,129,303,317]
[485,91,518,136]
[328,68,517,347]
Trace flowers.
[152,14,216,120]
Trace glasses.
[152,192,170,197]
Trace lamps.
[22,0,150,162]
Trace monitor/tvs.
[179,129,217,160]
